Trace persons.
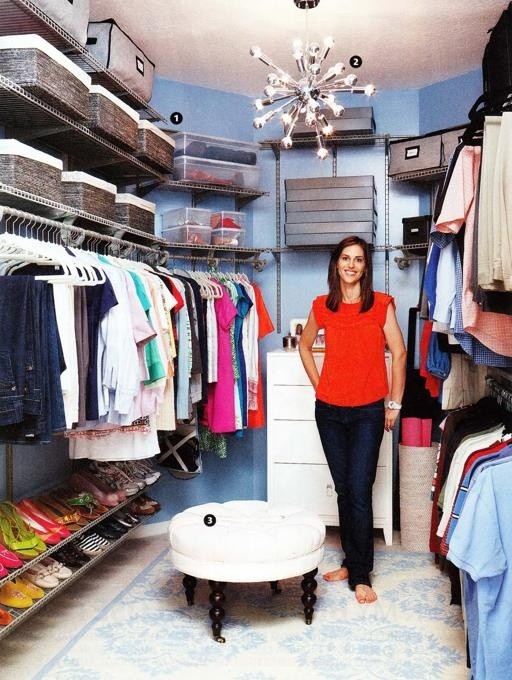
[296,236,410,606]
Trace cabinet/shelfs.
[264,346,396,546]
[1,478,154,639]
[263,134,449,255]
[0,0,171,270]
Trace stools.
[169,498,328,642]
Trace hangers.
[172,253,257,298]
[0,204,107,288]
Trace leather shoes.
[0,461,163,628]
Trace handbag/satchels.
[155,427,204,474]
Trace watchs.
[387,401,402,411]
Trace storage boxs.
[32,1,90,46]
[1,33,95,119]
[112,192,157,235]
[84,17,156,103]
[160,31,265,248]
[0,137,64,207]
[80,82,143,153]
[59,170,117,224]
[133,117,176,172]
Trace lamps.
[248,0,378,160]
